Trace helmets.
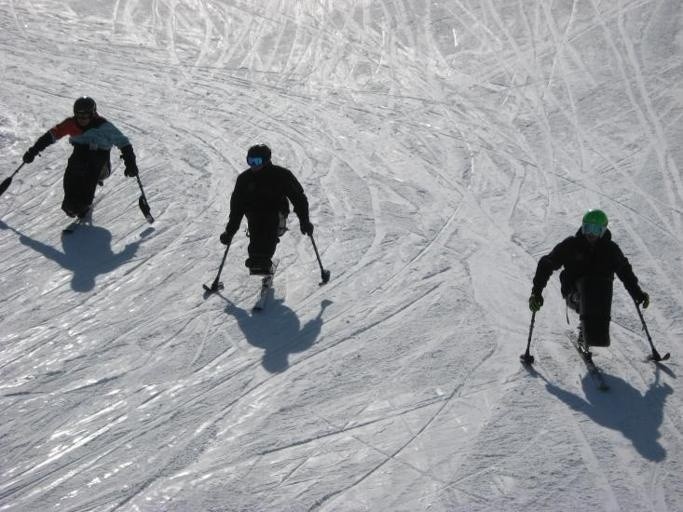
[581,208,609,235]
[73,96,97,115]
[247,143,271,161]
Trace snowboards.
[62,188,113,232]
[254,259,280,310]
[564,330,608,390]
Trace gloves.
[299,214,315,236]
[635,288,649,308]
[219,231,232,244]
[122,160,139,176]
[22,146,38,164]
[528,287,544,313]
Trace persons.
[218,143,314,269]
[20,95,138,217]
[527,209,649,348]
